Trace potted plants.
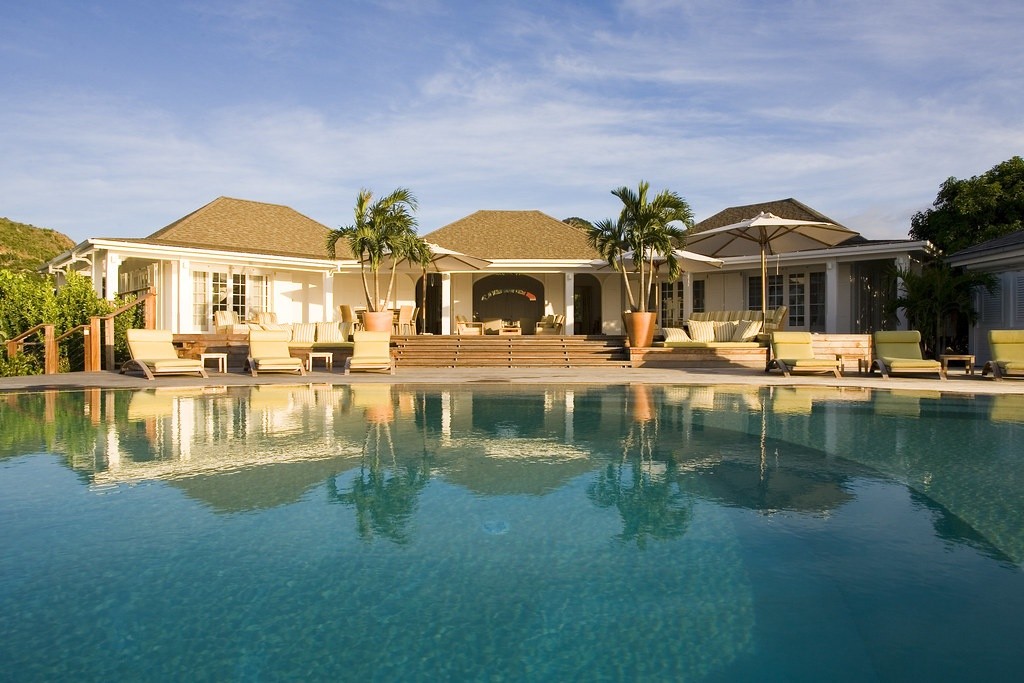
[325,187,433,335]
[586,179,695,347]
[327,403,432,545]
[588,383,695,551]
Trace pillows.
[713,319,739,341]
[732,319,763,342]
[688,319,717,342]
[248,321,353,342]
[659,327,693,342]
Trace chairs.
[982,330,1024,382]
[535,312,564,334]
[250,311,277,324]
[215,310,249,342]
[244,329,307,377]
[765,331,843,377]
[869,330,947,380]
[119,328,209,380]
[344,329,395,375]
[339,305,420,335]
[455,314,486,335]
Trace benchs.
[663,305,788,346]
[289,341,353,366]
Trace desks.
[835,353,868,376]
[195,352,227,373]
[939,353,975,377]
[355,308,401,335]
[498,326,521,334]
[306,351,333,371]
